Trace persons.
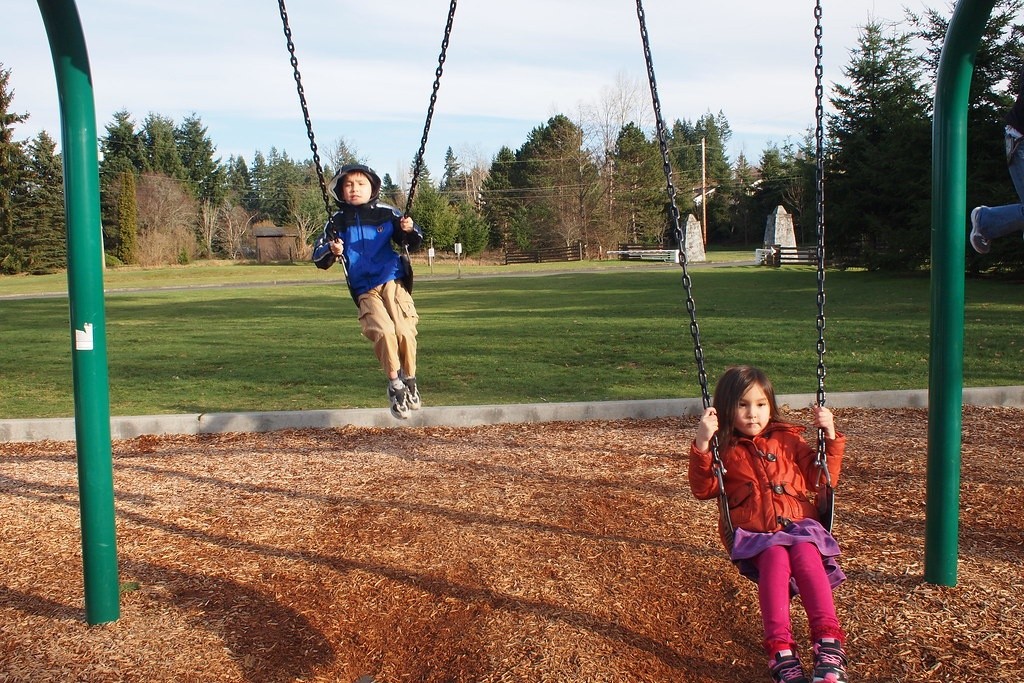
[689,366,849,683]
[312,164,423,419]
[970,68,1024,254]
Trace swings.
[636,0,839,591]
[274,0,460,307]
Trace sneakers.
[386,383,411,420]
[768,649,809,683]
[812,638,848,683]
[398,372,421,410]
